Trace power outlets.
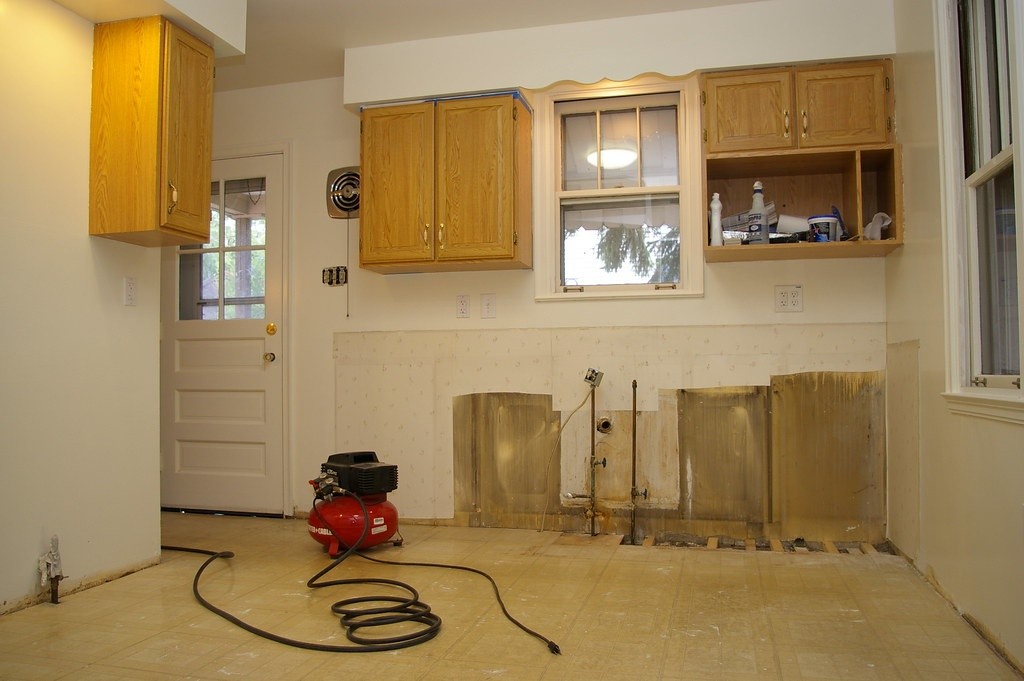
[480,293,496,319]
[774,284,804,313]
[456,294,471,319]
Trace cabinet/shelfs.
[701,59,904,264]
[359,90,535,275]
[90,16,216,249]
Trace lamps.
[587,139,639,170]
